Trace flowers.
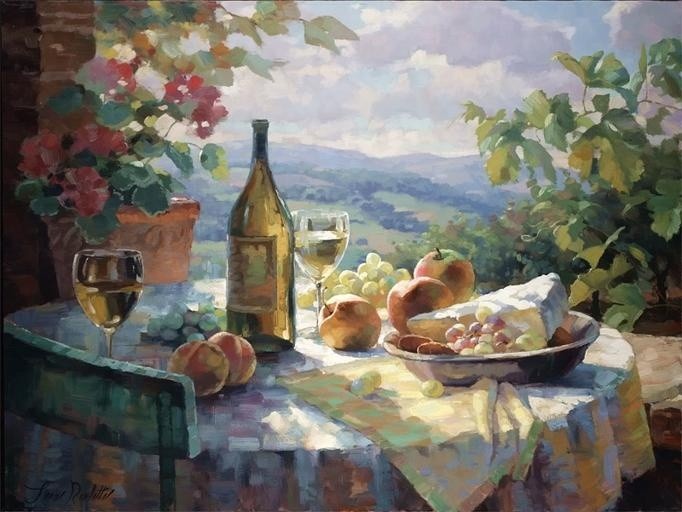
[17,55,231,247]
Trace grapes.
[444,308,546,359]
[418,379,444,397]
[350,369,381,396]
[297,253,412,311]
[145,298,228,350]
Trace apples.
[316,286,382,351]
[413,245,475,304]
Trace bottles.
[227,117,297,355]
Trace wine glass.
[72,249,144,359]
[288,209,351,339]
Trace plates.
[383,305,600,387]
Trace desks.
[2,275,637,511]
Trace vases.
[39,196,203,299]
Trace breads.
[405,272,570,342]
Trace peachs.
[166,332,256,400]
[386,276,454,335]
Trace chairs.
[2,318,201,511]
